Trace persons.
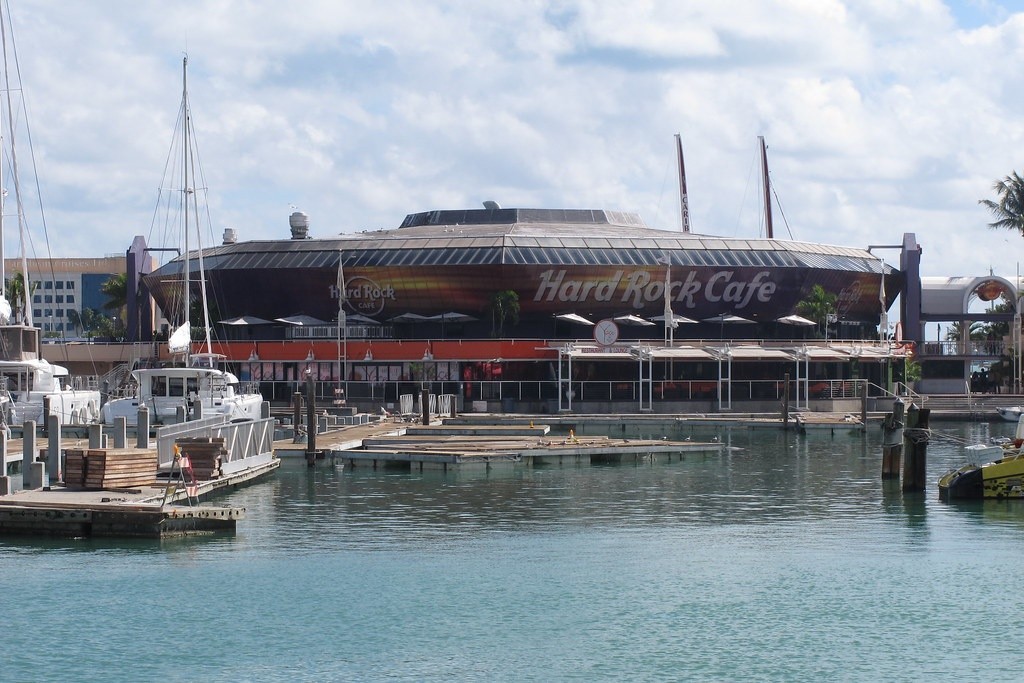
[972,368,1002,394]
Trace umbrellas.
[385,313,430,339]
[429,312,481,339]
[330,315,382,337]
[275,314,332,340]
[549,310,818,340]
[216,316,282,340]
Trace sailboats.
[1,0,265,453]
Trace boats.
[938,414,1024,500]
[996,406,1024,423]
